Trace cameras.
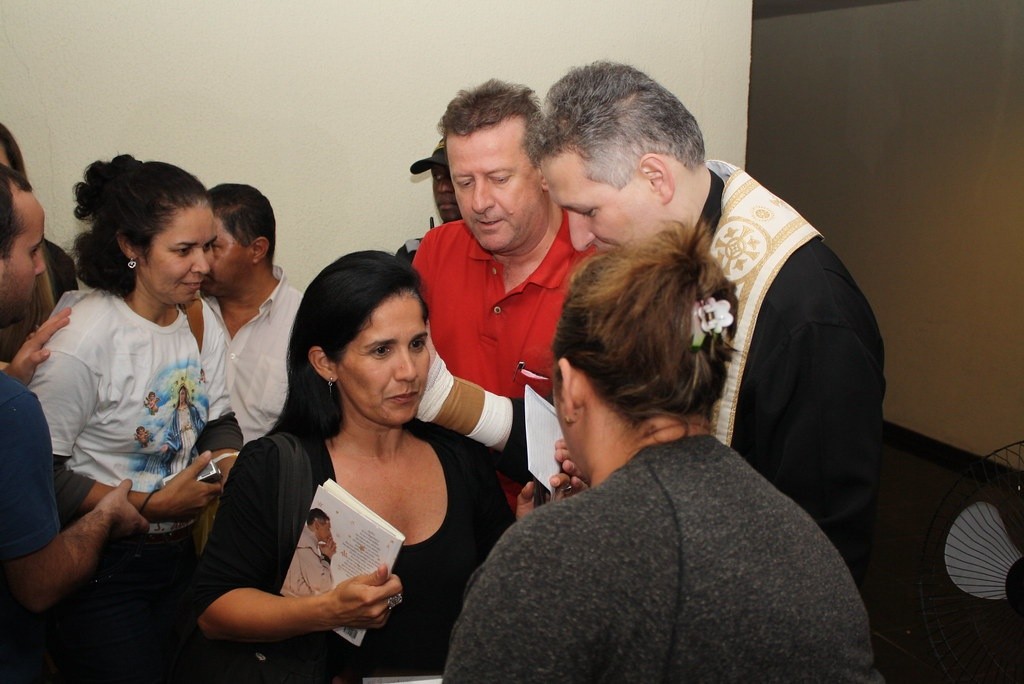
[161,460,223,484]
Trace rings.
[388,594,403,609]
[562,487,572,495]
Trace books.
[281,478,405,646]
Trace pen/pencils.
[513,361,525,382]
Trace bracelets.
[140,489,160,514]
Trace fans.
[918,441,1024,684]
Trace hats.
[410,138,449,175]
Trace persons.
[0,122,246,683]
[280,509,337,598]
[415,60,884,588]
[191,250,592,683]
[412,82,600,400]
[445,224,882,683]
[397,138,462,262]
[199,184,305,443]
